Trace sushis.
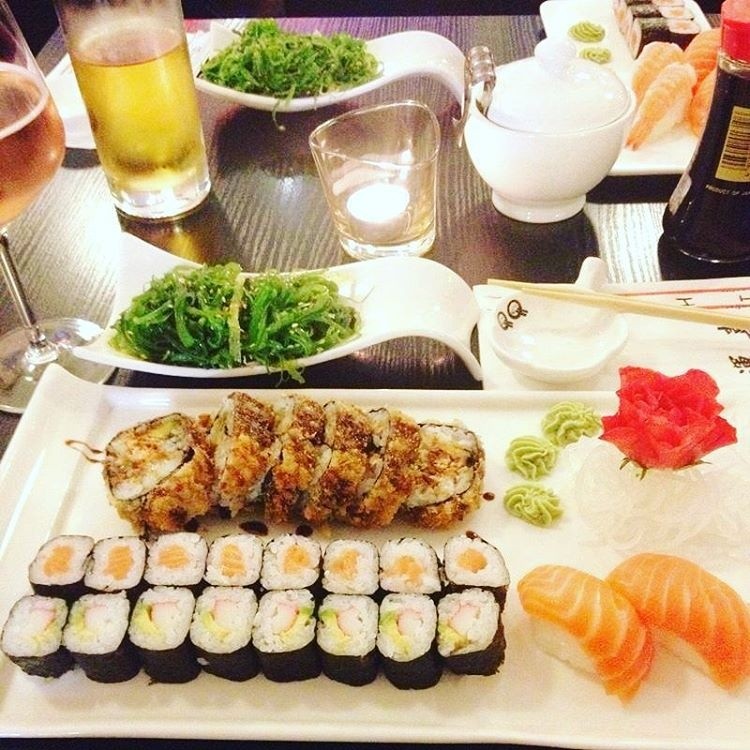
[189,583,261,685]
[439,522,513,613]
[434,589,507,683]
[662,20,701,48]
[140,531,205,591]
[332,398,420,530]
[687,62,718,138]
[678,26,722,93]
[0,594,77,680]
[203,392,274,513]
[317,529,379,601]
[83,533,147,600]
[376,527,446,598]
[60,591,139,685]
[669,6,696,24]
[126,586,203,687]
[617,7,658,33]
[627,20,668,55]
[202,531,263,594]
[401,418,485,534]
[517,561,653,702]
[628,39,685,107]
[608,0,651,16]
[620,61,697,155]
[602,549,749,690]
[376,587,445,693]
[98,408,216,532]
[300,397,371,525]
[258,532,324,595]
[250,588,325,688]
[315,584,378,691]
[23,532,92,600]
[262,393,329,520]
[650,0,680,14]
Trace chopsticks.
[487,279,749,330]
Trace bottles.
[659,1,749,278]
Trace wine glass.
[0,0,119,417]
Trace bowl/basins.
[489,259,631,381]
[475,37,637,225]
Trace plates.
[472,276,750,392]
[539,1,722,177]
[1,362,747,750]
[79,232,485,385]
[185,21,469,116]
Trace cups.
[54,0,210,217]
[306,98,441,261]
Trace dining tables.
[0,15,750,749]
